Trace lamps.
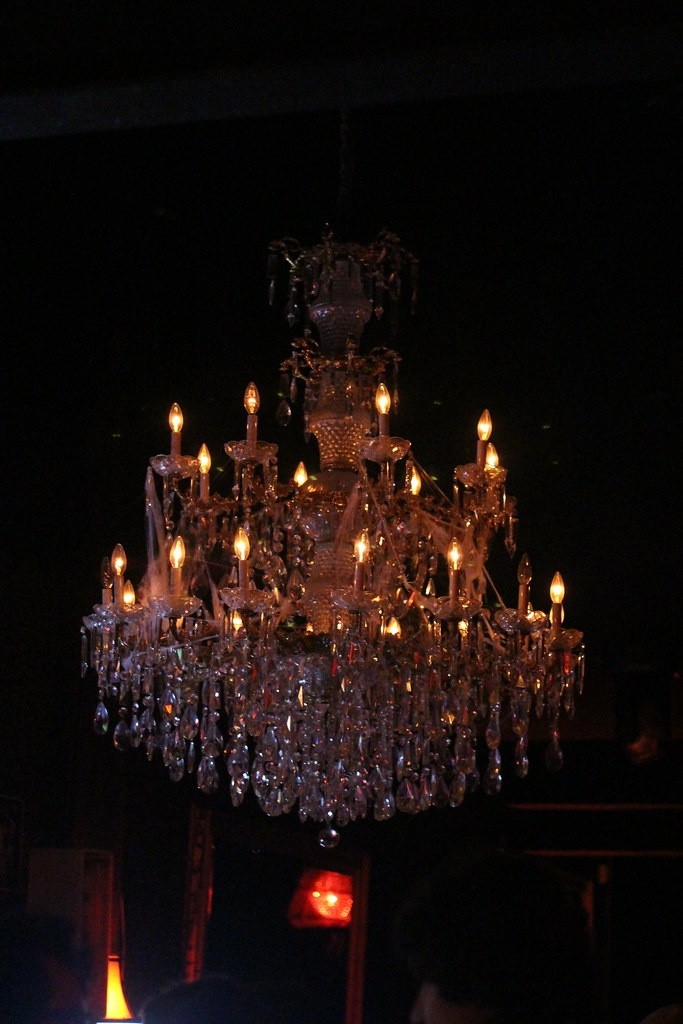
[71,229,589,849]
